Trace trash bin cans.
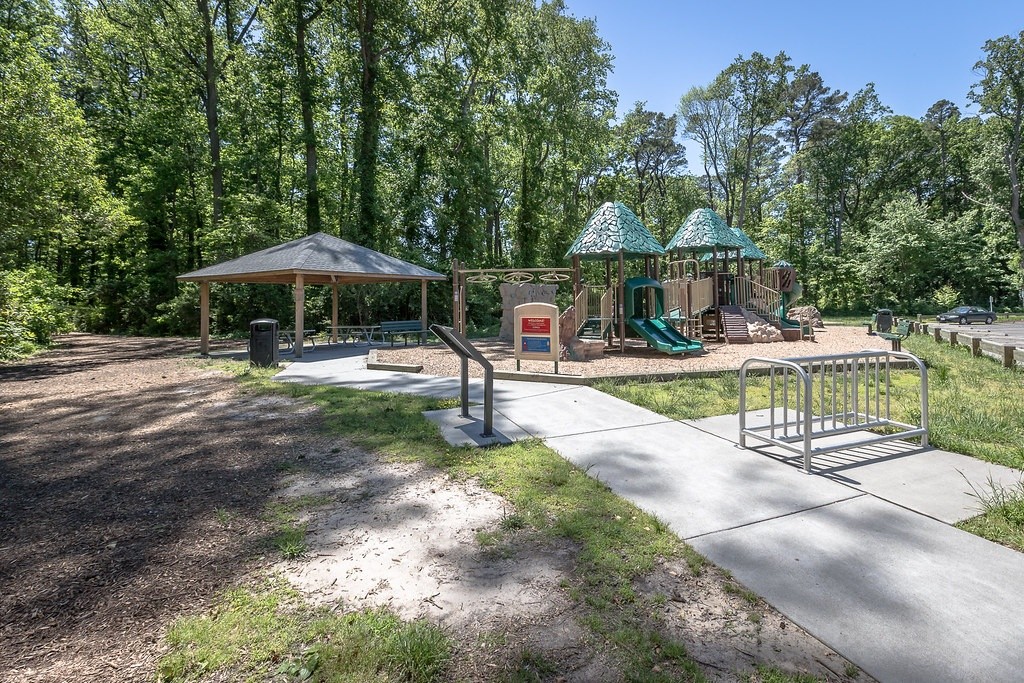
[877,309,893,332]
[250,318,279,367]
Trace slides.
[762,282,814,334]
[629,317,704,356]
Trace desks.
[246,330,316,354]
[327,326,386,348]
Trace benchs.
[878,321,909,359]
[862,313,877,335]
[380,319,431,346]
[326,331,388,335]
[278,336,321,344]
